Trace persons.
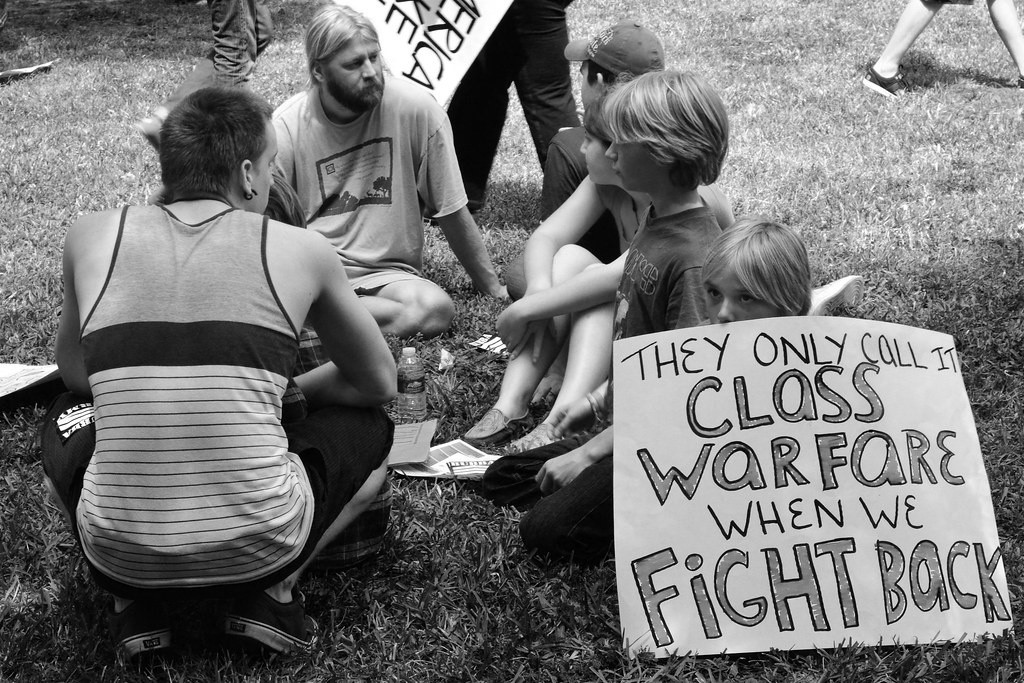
[862,0,1024,100]
[135,0,275,151]
[430,0,581,227]
[268,4,509,336]
[37,88,398,653]
[461,20,866,564]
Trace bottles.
[397,346,427,422]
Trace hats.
[564,22,664,79]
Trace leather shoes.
[504,423,565,453]
[464,407,536,448]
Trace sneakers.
[105,593,172,662]
[1018,78,1024,92]
[862,64,911,98]
[224,590,319,655]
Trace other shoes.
[422,204,438,225]
[138,107,168,150]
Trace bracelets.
[586,392,600,412]
[592,390,606,411]
